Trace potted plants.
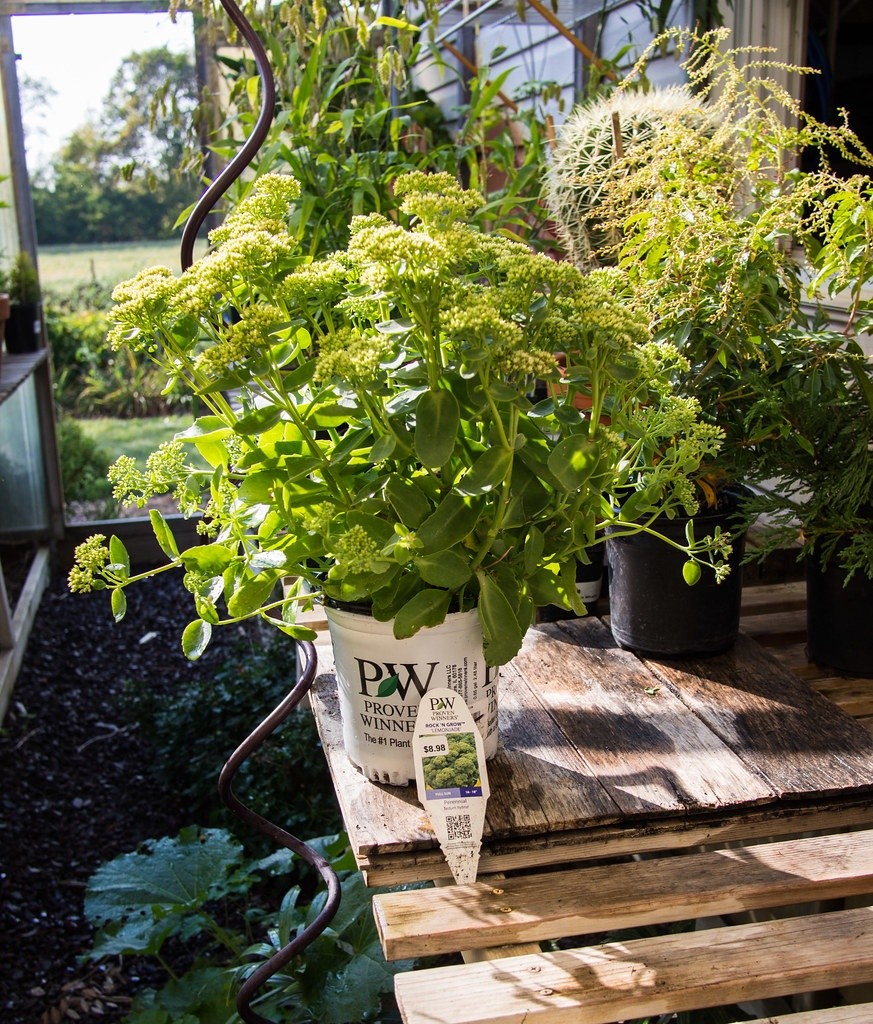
[75,2,872,786]
